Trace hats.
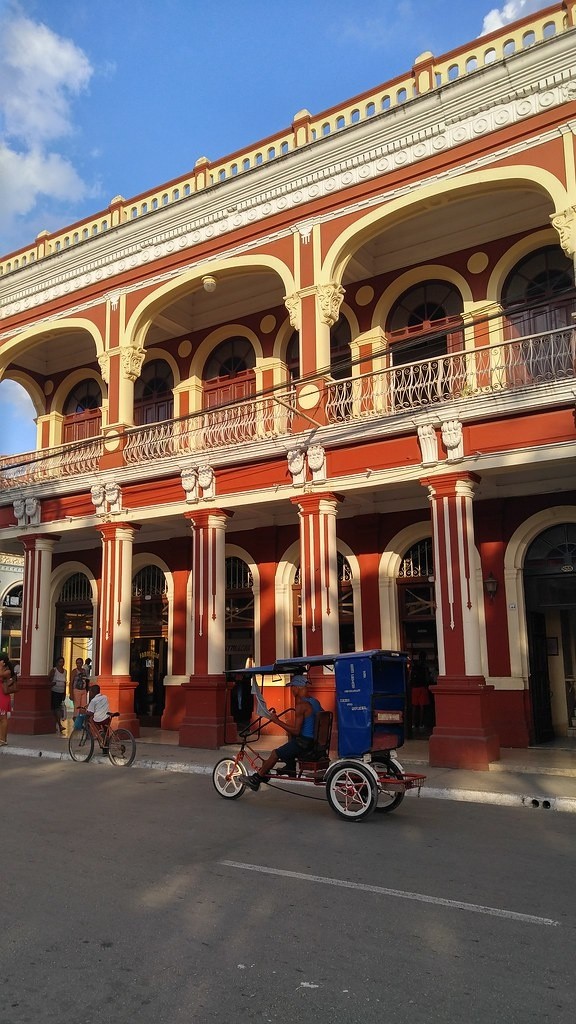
[286,675,312,686]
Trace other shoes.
[101,752,108,757]
[59,725,66,732]
[99,731,105,738]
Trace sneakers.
[277,760,296,777]
[239,773,263,792]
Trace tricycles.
[213,650,428,821]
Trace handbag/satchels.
[74,712,87,731]
[2,669,18,695]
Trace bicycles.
[67,707,136,767]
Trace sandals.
[0,740,7,747]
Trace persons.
[81,685,111,756]
[69,658,88,718]
[410,651,433,729]
[230,654,255,744]
[82,657,92,695]
[49,657,67,731]
[0,652,20,746]
[240,675,323,792]
